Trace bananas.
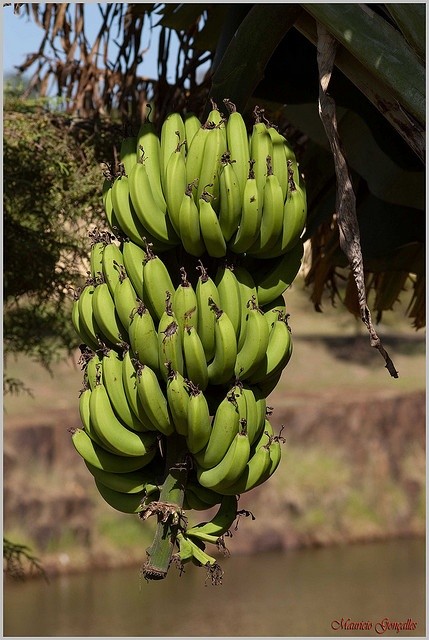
[65,99,311,580]
